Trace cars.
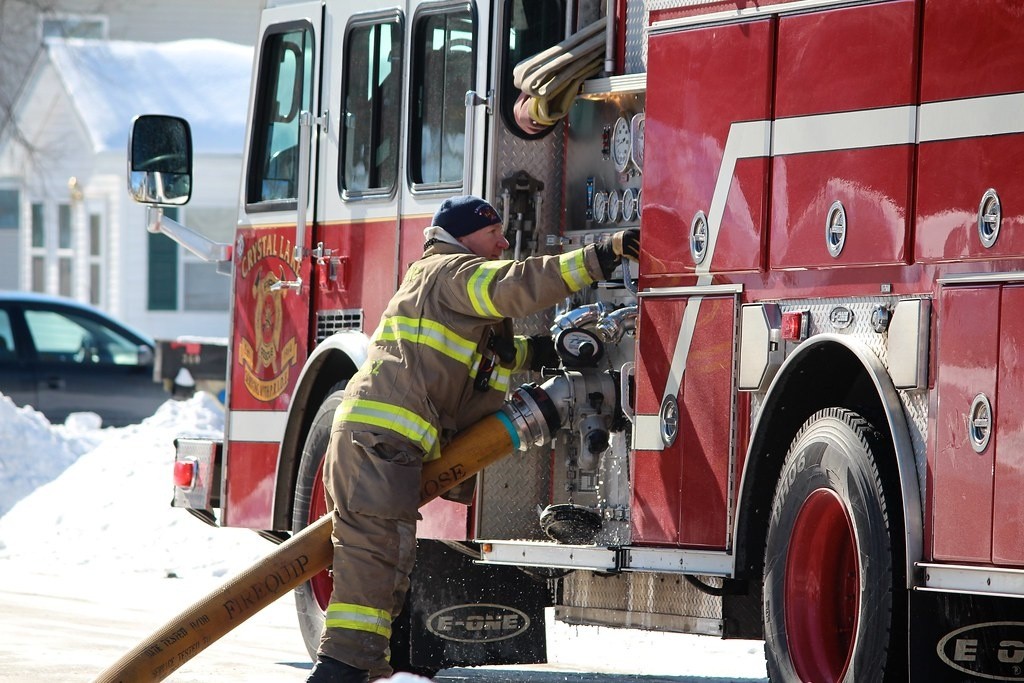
[0,290,224,430]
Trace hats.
[431,195,503,238]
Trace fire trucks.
[123,0,1024,683]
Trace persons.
[305,195,641,683]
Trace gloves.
[529,333,559,372]
[613,225,641,264]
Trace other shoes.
[306,656,369,683]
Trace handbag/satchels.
[347,423,425,518]
